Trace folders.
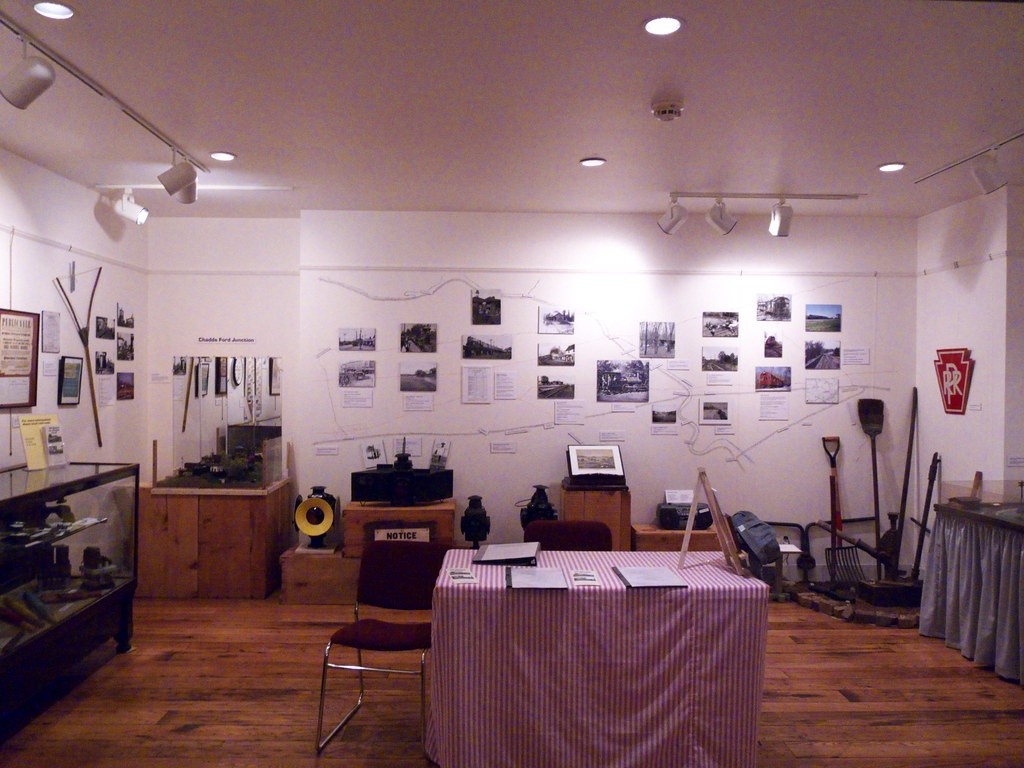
[472,542,540,567]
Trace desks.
[918,503,1024,689]
[632,524,723,550]
[425,550,772,768]
[280,498,457,605]
[557,488,632,550]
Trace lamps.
[113,187,150,226]
[656,195,690,236]
[156,149,200,196]
[0,33,57,110]
[175,155,197,204]
[704,197,738,235]
[768,200,795,237]
[972,147,1010,195]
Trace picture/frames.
[699,394,733,427]
[58,355,82,406]
[567,444,625,476]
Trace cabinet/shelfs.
[132,477,293,599]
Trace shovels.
[822,436,866,589]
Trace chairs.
[523,520,612,552]
[315,540,452,755]
